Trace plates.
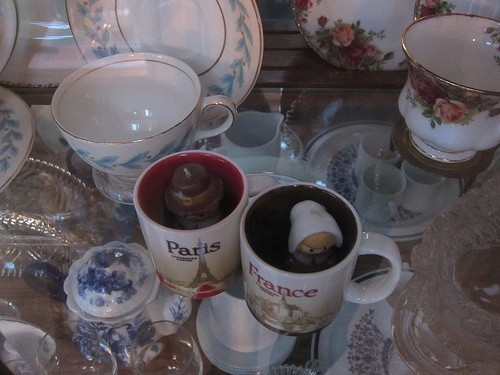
[0,0,36,197]
[310,266,438,375]
[64,0,265,122]
[196,297,297,375]
[290,0,500,71]
[91,166,134,205]
[296,120,466,241]
[193,109,303,176]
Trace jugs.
[207,110,285,175]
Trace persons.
[284,201,343,274]
[147,163,238,230]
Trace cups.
[132,150,280,299]
[355,132,444,223]
[239,181,402,336]
[49,52,236,177]
[398,13,500,163]
[34,242,204,375]
[207,280,279,353]
